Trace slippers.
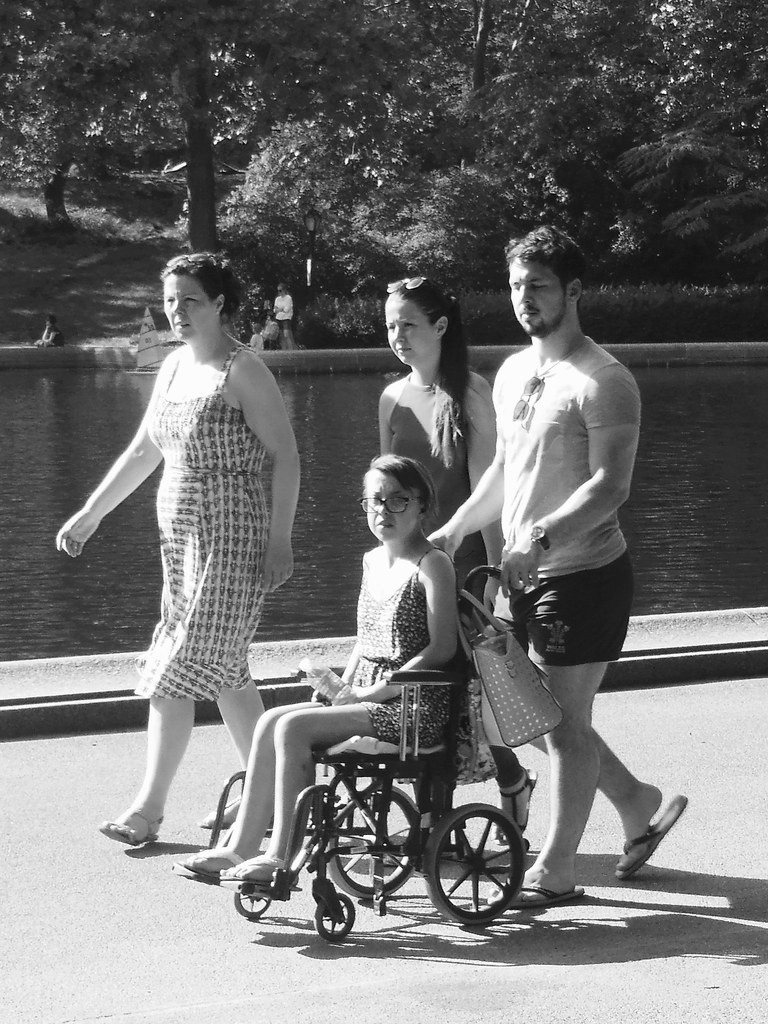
[219,854,304,892]
[614,794,687,879]
[488,881,585,910]
[176,847,245,877]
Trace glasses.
[512,374,543,422]
[361,497,422,513]
[386,276,454,301]
[165,254,219,265]
[277,290,283,292]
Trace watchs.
[527,525,552,553]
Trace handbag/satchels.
[454,588,566,747]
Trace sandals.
[495,767,538,846]
[200,797,239,830]
[100,808,164,845]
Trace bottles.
[297,659,351,702]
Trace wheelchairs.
[170,562,528,942]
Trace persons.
[42,316,64,347]
[172,450,456,881]
[374,278,537,851]
[55,254,300,847]
[244,281,296,352]
[418,224,662,903]
